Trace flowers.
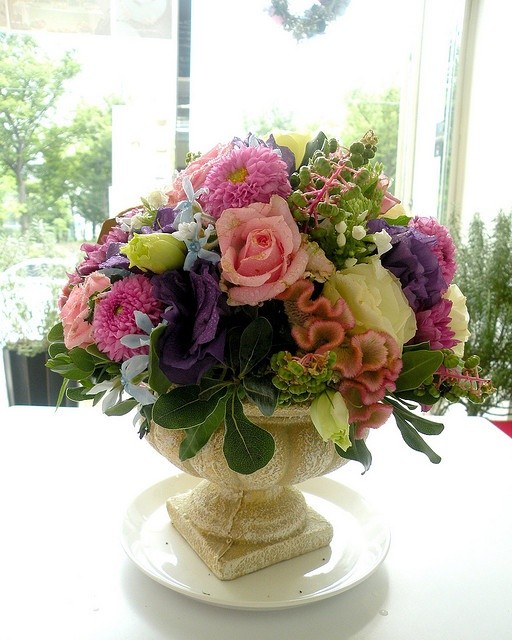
[41,124,494,479]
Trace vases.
[140,394,370,584]
